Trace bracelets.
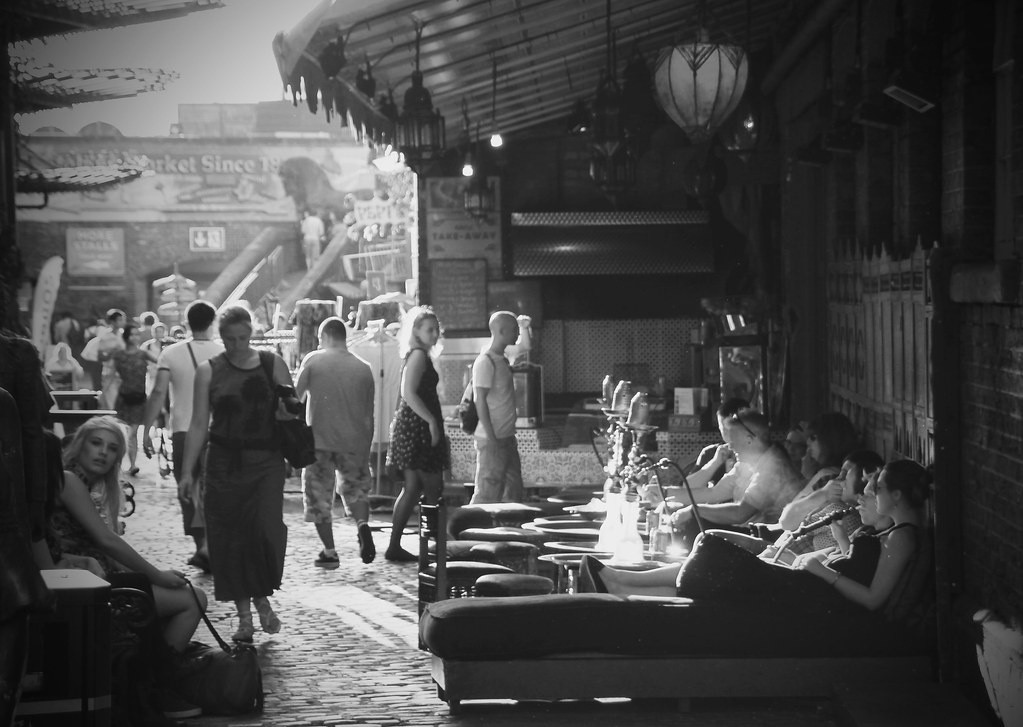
[831,571,841,585]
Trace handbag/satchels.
[260,349,317,469]
[165,639,264,715]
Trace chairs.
[0,333,173,727]
[420,457,950,713]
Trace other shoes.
[386,548,418,562]
[358,522,376,563]
[315,552,339,567]
[192,553,210,572]
[578,555,607,595]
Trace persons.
[385,306,450,561]
[295,317,376,567]
[178,308,299,641]
[469,311,523,504]
[143,303,227,572]
[45,308,293,477]
[578,398,935,646]
[0,387,207,727]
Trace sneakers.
[150,687,202,718]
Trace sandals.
[253,596,281,634]
[232,612,252,641]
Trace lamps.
[397,0,758,225]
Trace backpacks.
[459,353,496,434]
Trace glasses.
[732,412,757,438]
[874,484,889,494]
